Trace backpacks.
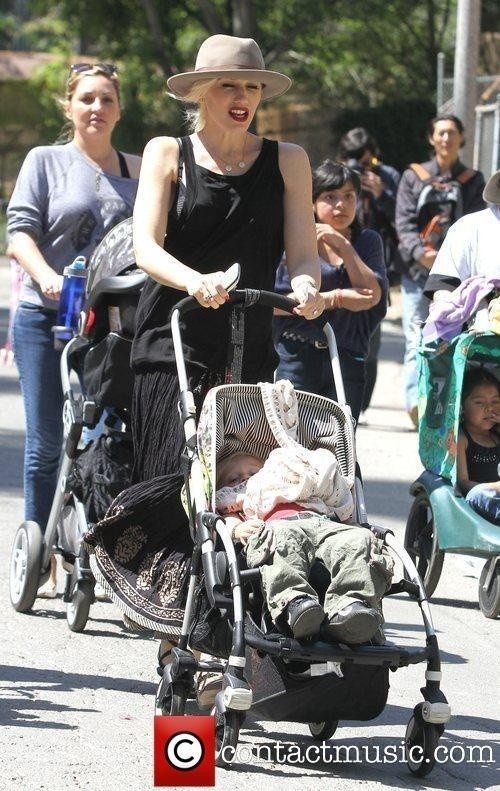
[409,163,478,281]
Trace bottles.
[54,256,89,342]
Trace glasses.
[68,63,117,77]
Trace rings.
[312,309,318,314]
[205,294,212,300]
[47,285,54,294]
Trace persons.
[219,447,386,644]
[457,366,499,525]
[334,127,400,423]
[123,33,322,679]
[396,113,487,427]
[422,172,499,301]
[276,160,388,423]
[5,61,142,597]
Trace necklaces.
[70,137,116,190]
[202,127,249,172]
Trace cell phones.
[222,263,242,293]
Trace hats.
[168,35,292,105]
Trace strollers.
[9,216,137,635]
[151,287,451,781]
[402,270,499,620]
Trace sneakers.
[282,596,383,643]
